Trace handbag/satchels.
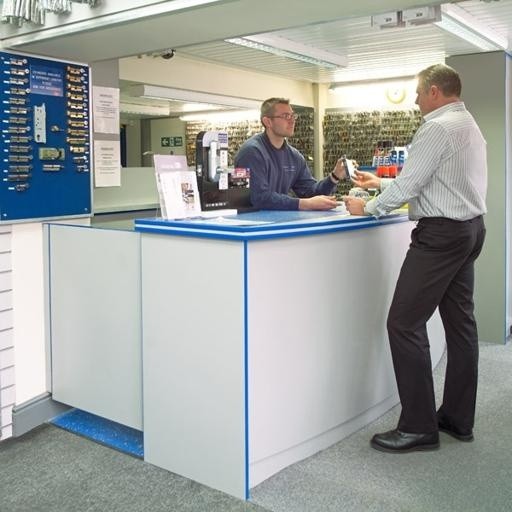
[332,171,341,181]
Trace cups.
[270,113,298,119]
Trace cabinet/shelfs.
[372,141,405,178]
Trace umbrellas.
[438,424,473,440]
[371,428,440,453]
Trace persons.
[343,62,488,454]
[234,98,359,215]
